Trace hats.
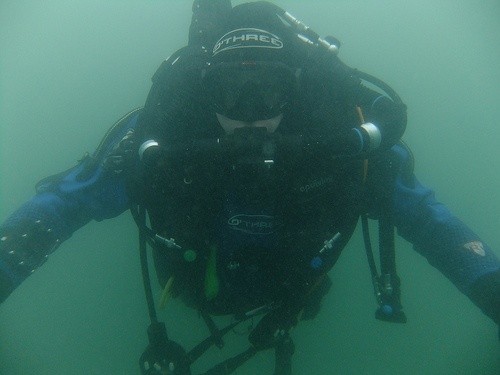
[200,4,300,66]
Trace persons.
[0,0,500,330]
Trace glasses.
[205,59,294,121]
